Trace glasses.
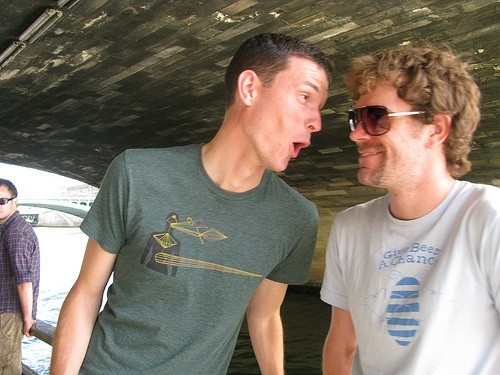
[0,197,15,205]
[345,105,429,137]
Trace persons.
[319,43,500,375]
[47,32,335,375]
[0,179,40,375]
